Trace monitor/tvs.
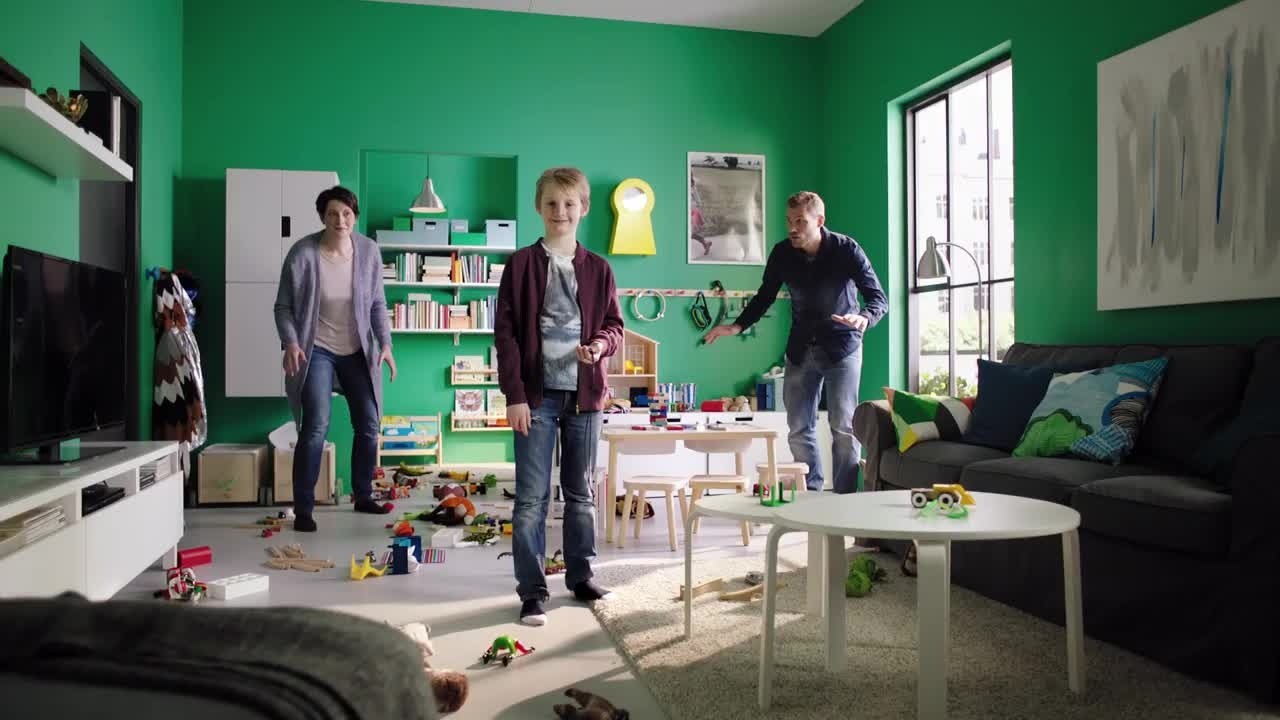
[0,245,129,463]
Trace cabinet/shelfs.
[374,242,517,346]
[449,365,514,431]
[226,167,340,397]
[603,329,659,410]
[0,440,184,606]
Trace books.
[370,244,515,449]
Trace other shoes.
[704,241,712,255]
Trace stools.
[553,461,809,552]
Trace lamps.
[409,154,446,213]
[918,236,982,358]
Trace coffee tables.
[684,490,1083,720]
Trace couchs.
[851,343,1280,694]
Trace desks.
[601,427,782,548]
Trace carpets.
[587,553,1280,720]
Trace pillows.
[882,357,1169,464]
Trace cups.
[177,545,212,568]
[658,382,676,412]
[648,392,668,427]
[680,383,697,412]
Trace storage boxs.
[273,442,335,502]
[376,207,517,247]
[755,376,786,411]
[196,442,269,503]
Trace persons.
[703,188,890,493]
[494,165,616,626]
[272,184,398,534]
